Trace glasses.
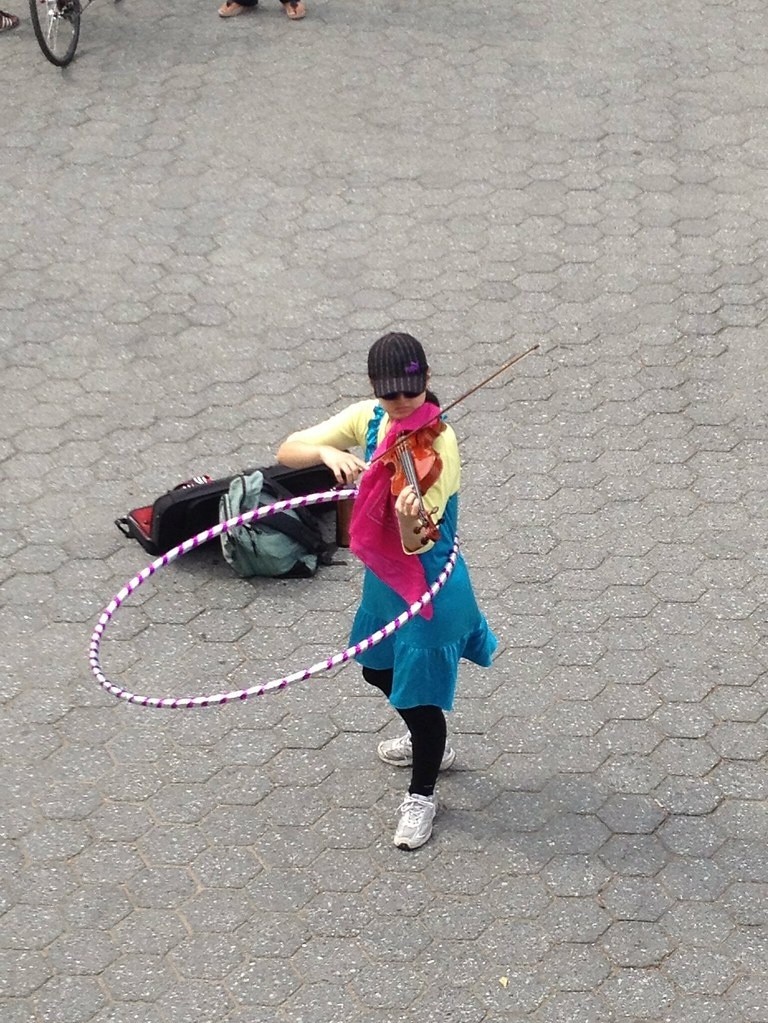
[381,390,425,400]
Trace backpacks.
[219,470,347,580]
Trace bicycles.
[28,0,121,68]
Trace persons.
[275,333,499,851]
[218,0,307,20]
[0,10,20,32]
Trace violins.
[381,416,449,545]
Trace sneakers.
[393,785,438,851]
[377,730,456,771]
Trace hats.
[368,332,427,398]
